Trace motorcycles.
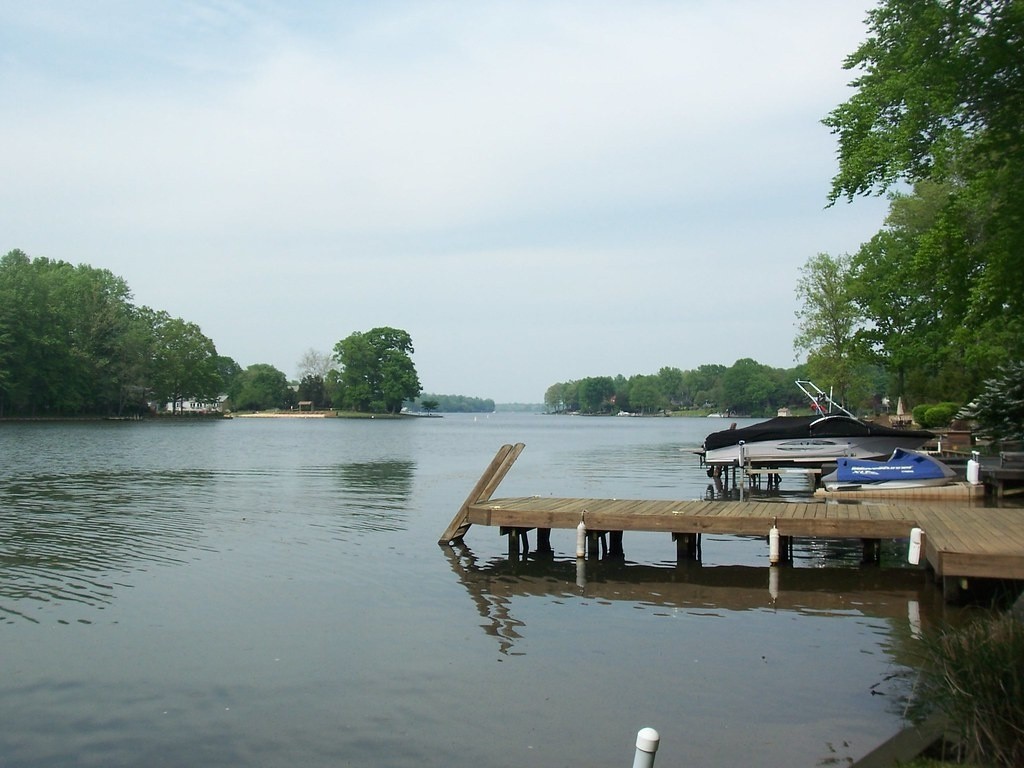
[821,447,957,492]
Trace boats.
[705,414,936,467]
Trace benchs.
[998,451,1024,468]
